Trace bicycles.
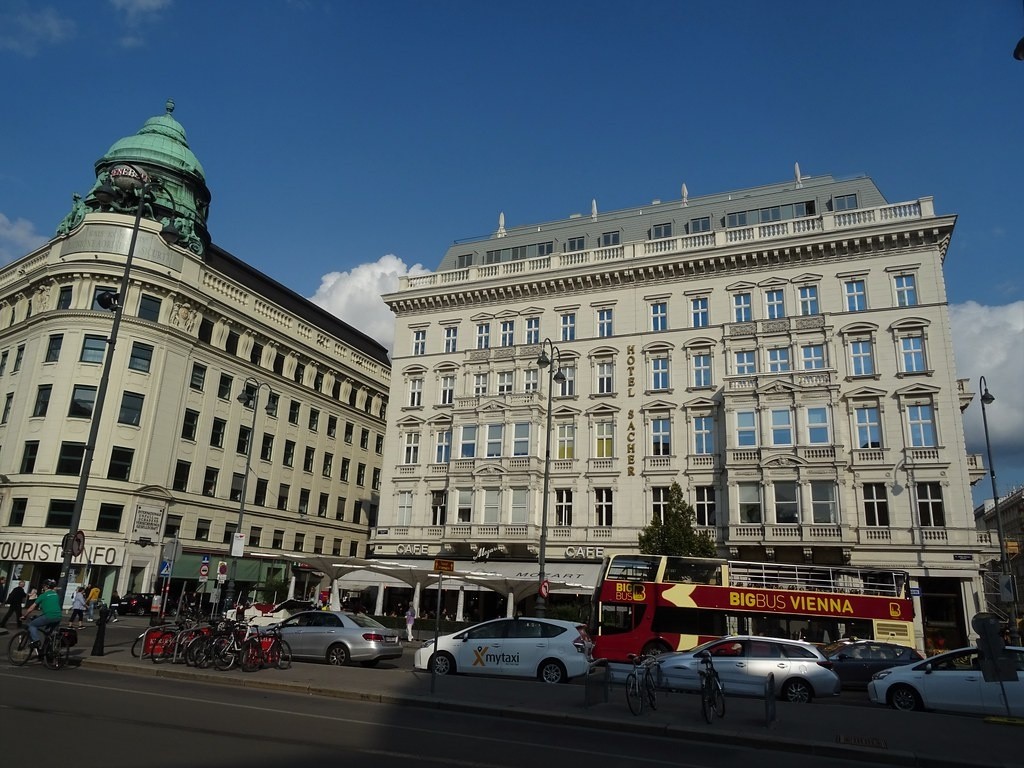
[623,652,666,716]
[7,615,70,670]
[697,650,725,723]
[130,607,292,671]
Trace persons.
[35,287,51,313]
[67,584,103,630]
[170,302,197,333]
[0,576,6,606]
[61,192,84,234]
[406,602,415,641]
[19,579,62,665]
[107,591,121,623]
[389,601,447,620]
[176,208,195,243]
[0,580,32,628]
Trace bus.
[576,553,926,667]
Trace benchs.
[368,615,483,641]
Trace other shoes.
[29,640,41,646]
[0,623,7,628]
[67,626,75,630]
[78,625,86,630]
[87,618,94,621]
[113,619,118,623]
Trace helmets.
[44,579,56,587]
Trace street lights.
[56,161,182,631]
[224,377,276,617]
[535,337,566,637]
[978,375,1021,647]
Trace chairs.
[971,657,981,671]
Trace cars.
[641,635,842,704]
[866,644,1024,719]
[816,636,926,690]
[248,610,403,667]
[117,593,154,615]
[414,611,596,683]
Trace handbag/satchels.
[59,627,77,646]
